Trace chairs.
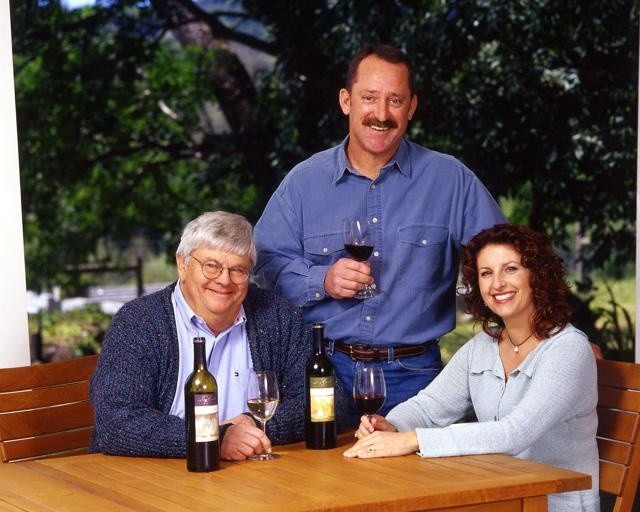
[0,352,99,463]
[599,355,639,510]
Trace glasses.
[195,259,250,285]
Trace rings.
[367,447,374,453]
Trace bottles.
[303,322,338,450]
[183,337,222,473]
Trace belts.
[323,340,435,361]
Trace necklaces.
[503,328,536,355]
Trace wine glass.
[353,366,386,421]
[342,216,378,300]
[247,370,280,461]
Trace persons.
[248,42,604,426]
[339,220,604,511]
[83,209,358,465]
